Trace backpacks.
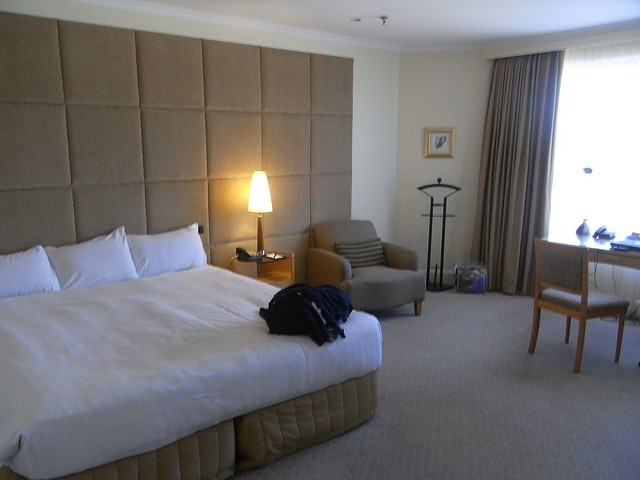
[259,283,353,346]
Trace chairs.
[527,237,630,374]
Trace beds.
[0,264,383,479]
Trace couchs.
[309,219,426,317]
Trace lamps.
[572,166,595,237]
[246,171,274,254]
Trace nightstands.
[231,251,295,287]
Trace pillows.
[126,221,210,277]
[1,245,62,295]
[46,226,138,290]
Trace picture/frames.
[424,128,456,158]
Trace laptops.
[610,232,640,251]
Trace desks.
[561,235,640,273]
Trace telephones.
[237,247,262,261]
